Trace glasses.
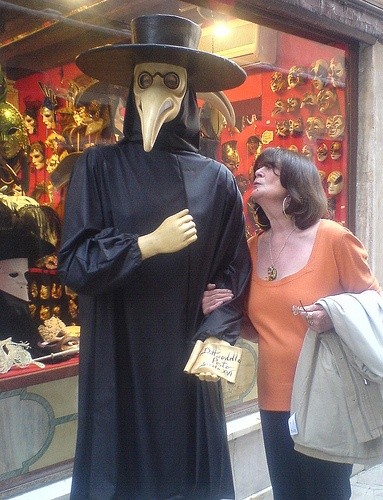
[291,299,309,316]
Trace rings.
[307,312,312,319]
[308,320,312,326]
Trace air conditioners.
[197,20,278,69]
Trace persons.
[201,147,382,500]
[56,14,251,500]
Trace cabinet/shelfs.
[0,338,260,500]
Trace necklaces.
[268,224,296,280]
